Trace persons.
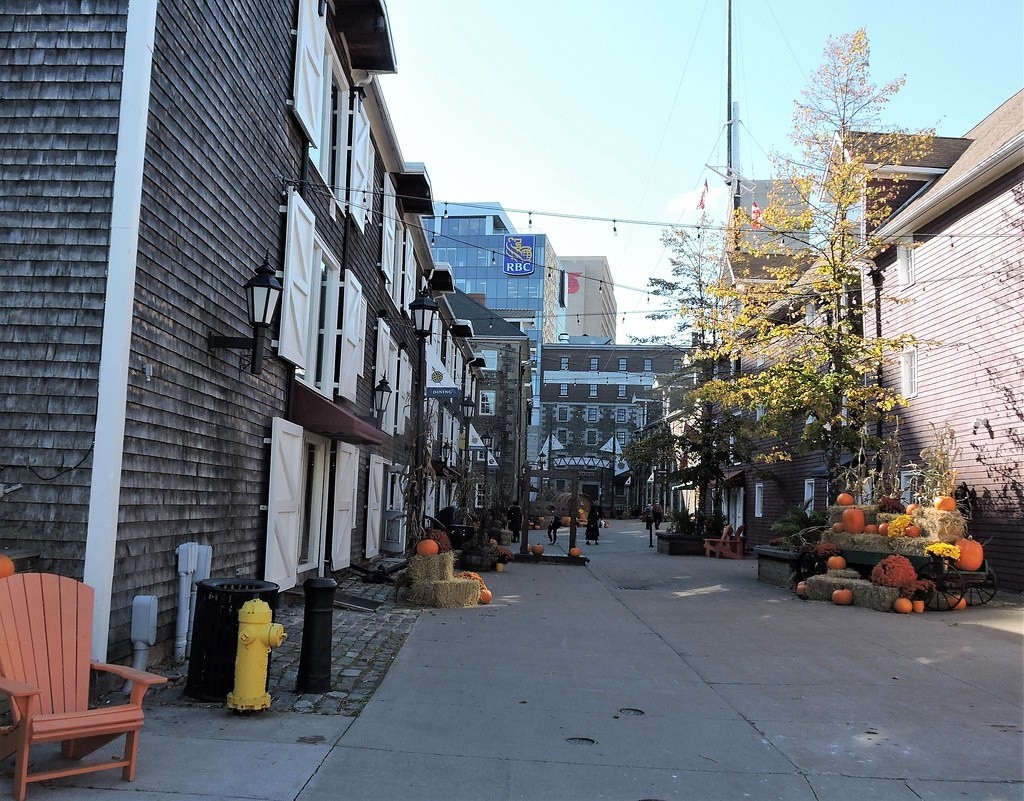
[597,503,604,520]
[652,501,664,530]
[547,512,561,546]
[507,499,522,543]
[585,505,600,545]
[644,503,654,529]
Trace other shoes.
[548,541,553,545]
[595,542,599,545]
[552,542,556,546]
[586,541,591,545]
[511,539,515,543]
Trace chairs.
[704,524,745,559]
[0,573,167,801]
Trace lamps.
[211,248,284,375]
[354,370,393,431]
[440,439,451,466]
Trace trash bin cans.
[186,578,279,702]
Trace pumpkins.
[528,543,545,555]
[798,581,807,595]
[530,514,611,529]
[828,554,846,569]
[0,552,14,579]
[479,589,493,605]
[570,548,581,556]
[832,493,956,536]
[954,534,983,572]
[948,594,966,610]
[417,539,439,555]
[832,589,853,605]
[894,595,913,613]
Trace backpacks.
[645,507,651,515]
[552,515,560,530]
